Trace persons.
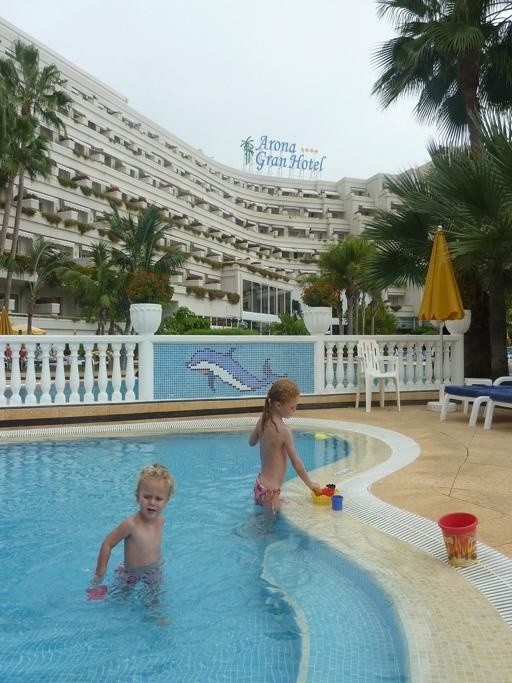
[89,463,176,604]
[248,378,323,525]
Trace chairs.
[355,340,401,413]
[440,376,512,429]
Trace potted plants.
[445,277,473,335]
[302,284,343,335]
[127,271,174,335]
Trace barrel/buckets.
[439,513,478,567]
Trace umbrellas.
[0,306,13,336]
[417,224,465,391]
